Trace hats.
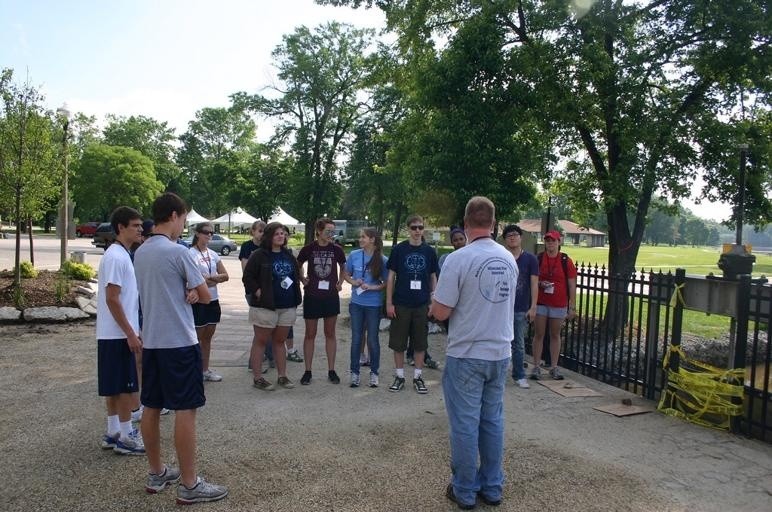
[543,228,562,241]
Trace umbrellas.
[262,206,305,228]
[185,208,210,227]
[212,206,261,227]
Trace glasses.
[198,230,215,236]
[504,232,521,239]
[408,224,426,231]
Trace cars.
[72,220,236,257]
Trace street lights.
[57,107,72,273]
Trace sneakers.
[514,377,530,389]
[445,479,476,511]
[203,367,222,382]
[99,432,122,449]
[478,479,503,507]
[529,366,541,379]
[248,348,440,395]
[176,478,231,504]
[549,367,563,379]
[113,432,149,456]
[130,410,144,423]
[159,406,172,417]
[147,463,182,494]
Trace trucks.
[333,219,371,247]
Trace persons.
[96,205,147,456]
[133,191,229,505]
[430,195,521,510]
